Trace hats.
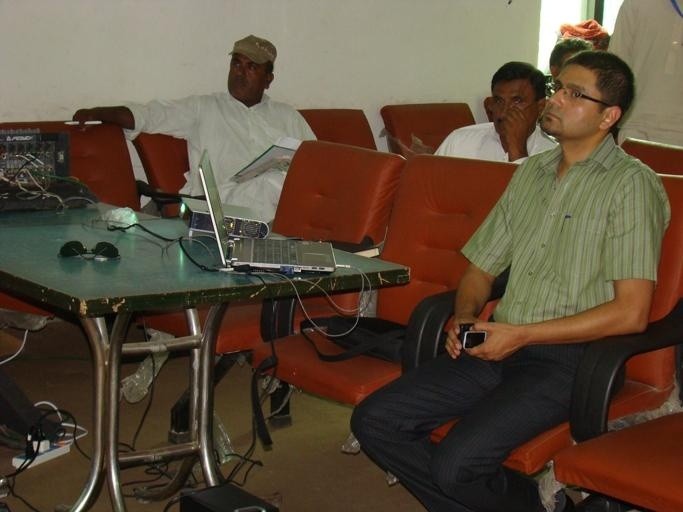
[227,34,277,66]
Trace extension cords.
[12,443,70,468]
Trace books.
[327,241,381,257]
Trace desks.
[2,199,412,512]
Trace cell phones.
[462,330,486,349]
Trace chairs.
[553,317,682,510]
[0,120,141,316]
[622,138,682,175]
[130,99,518,445]
[419,171,682,475]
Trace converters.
[33,440,50,453]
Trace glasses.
[56,240,121,263]
[543,75,614,108]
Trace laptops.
[197,151,337,276]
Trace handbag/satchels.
[298,314,410,366]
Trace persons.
[73,34,318,223]
[607,0,683,147]
[350,51,671,512]
[549,39,594,77]
[433,63,560,167]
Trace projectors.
[178,197,269,239]
